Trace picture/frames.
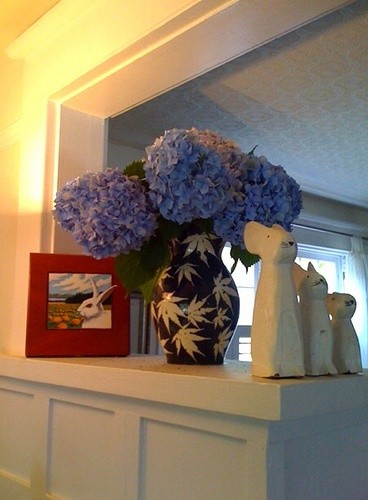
[25,253,134,358]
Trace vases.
[149,232,240,364]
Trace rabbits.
[77,277,117,329]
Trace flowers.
[53,126,304,304]
